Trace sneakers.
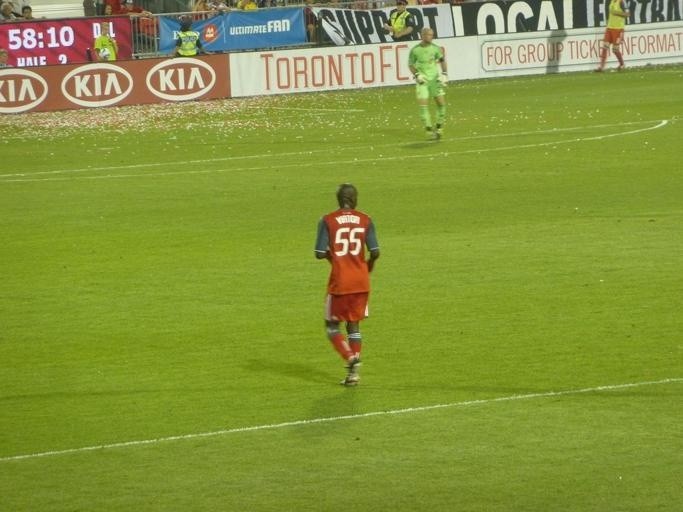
[427,125,445,142]
[340,356,364,386]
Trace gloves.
[414,72,429,85]
[436,72,448,84]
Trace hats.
[396,0,408,5]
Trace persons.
[383,1,416,41]
[117,0,152,58]
[593,0,631,73]
[408,27,448,138]
[304,7,317,42]
[344,2,368,9]
[1,2,16,66]
[275,1,285,6]
[191,1,258,53]
[168,15,212,59]
[314,184,379,386]
[101,3,113,15]
[1,49,14,67]
[14,5,46,20]
[94,22,118,62]
[260,1,277,7]
